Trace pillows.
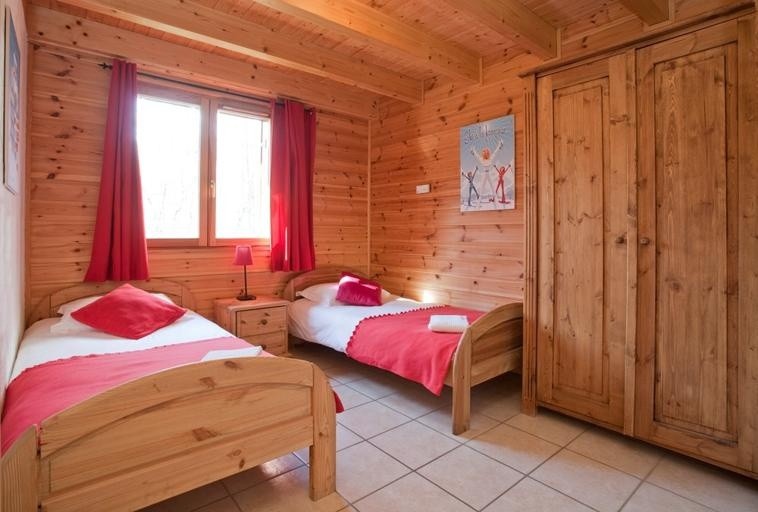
[296,282,392,308]
[335,271,383,307]
[50,292,195,333]
[71,281,186,339]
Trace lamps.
[232,244,257,301]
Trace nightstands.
[214,294,290,356]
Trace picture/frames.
[4,5,21,197]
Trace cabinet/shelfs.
[519,2,758,481]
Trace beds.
[0,280,344,512]
[284,267,523,435]
